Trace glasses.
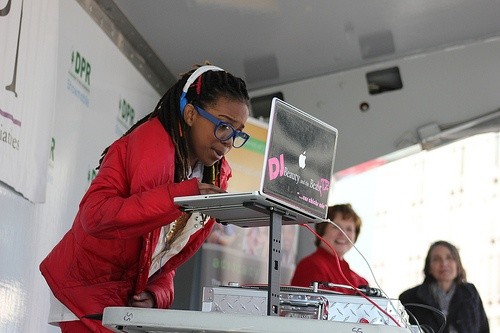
[195,106,250,148]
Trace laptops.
[174,97,338,228]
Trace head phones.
[179,65,225,122]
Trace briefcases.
[202,281,410,328]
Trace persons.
[292,203,371,295]
[39,61,250,333]
[398,241,490,333]
